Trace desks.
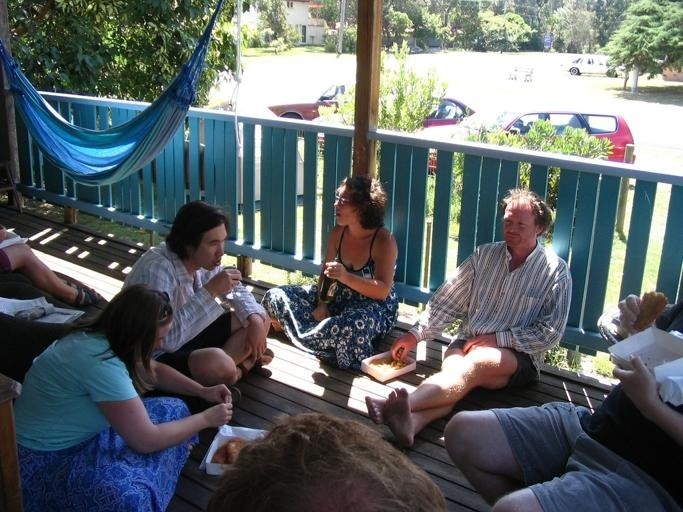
[0,374,24,512]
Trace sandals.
[238,348,273,377]
[66,281,101,307]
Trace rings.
[329,267,333,273]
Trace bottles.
[318,258,340,304]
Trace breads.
[211,438,245,465]
[633,291,667,331]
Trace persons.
[443,290,683,512]
[209,407,448,512]
[12,281,233,512]
[365,186,574,450]
[121,200,274,406]
[259,176,400,372]
[0,225,102,308]
[0,278,87,405]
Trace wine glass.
[222,263,241,300]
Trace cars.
[423,97,476,126]
[268,85,346,121]
[568,56,618,78]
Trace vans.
[499,111,634,163]
